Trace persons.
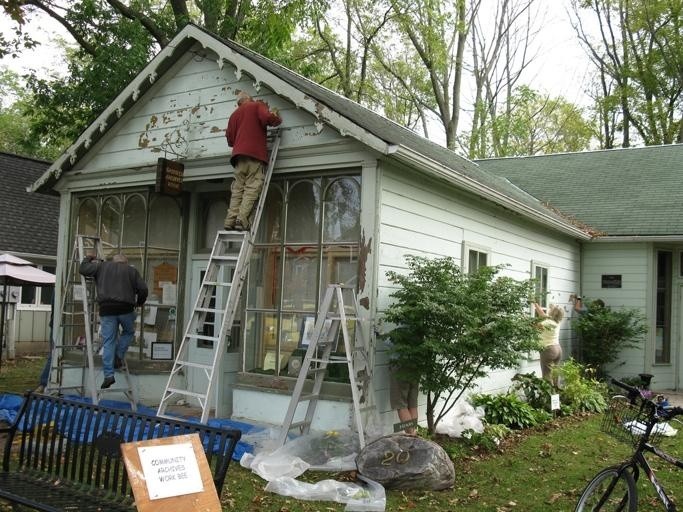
[78,249,148,390]
[222,92,282,232]
[532,300,565,387]
[375,320,422,436]
[572,294,606,363]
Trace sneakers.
[115,357,124,369]
[100,378,115,389]
[33,384,45,394]
[235,220,248,230]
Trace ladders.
[44,234,138,413]
[279,284,381,451]
[156,128,283,425]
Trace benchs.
[0,389,242,512]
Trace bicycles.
[573,376,683,511]
